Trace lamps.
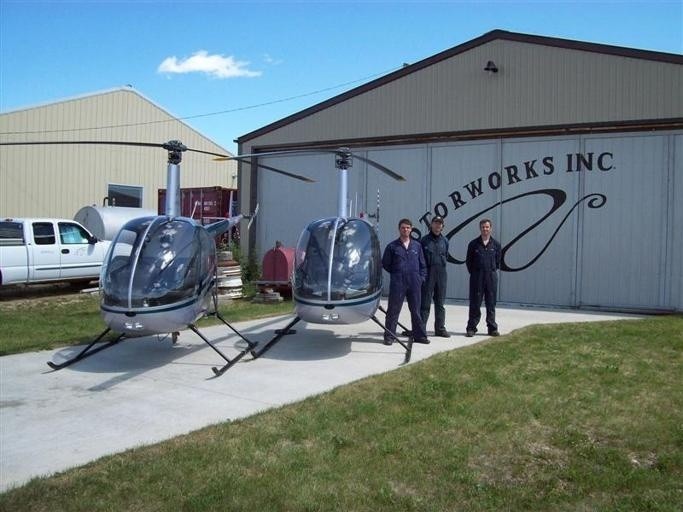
[484,61,499,77]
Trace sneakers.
[414,337,430,344]
[383,338,392,345]
[466,330,475,337]
[435,331,450,337]
[488,330,498,336]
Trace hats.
[432,216,443,223]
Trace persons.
[381,217,430,345]
[464,218,502,337]
[418,214,452,339]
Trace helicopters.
[0,139,318,376]
[212,143,420,364]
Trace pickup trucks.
[0,217,142,293]
